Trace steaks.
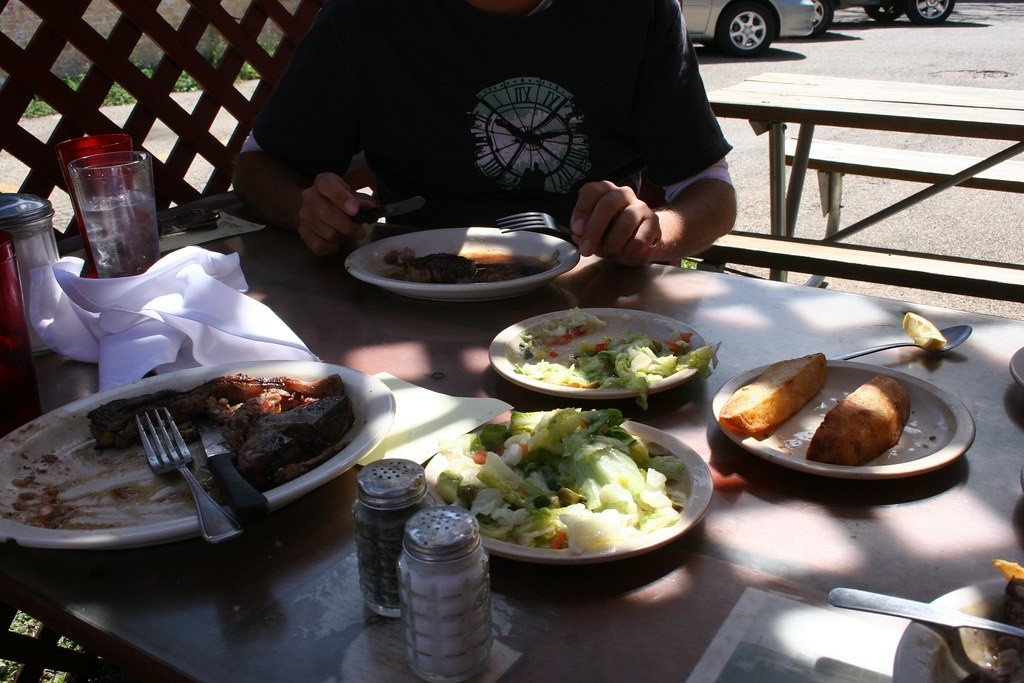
[225,389,354,485]
[403,251,514,284]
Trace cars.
[806,0,957,39]
[678,0,818,59]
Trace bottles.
[397,506,493,683]
[0,192,62,359]
[351,458,429,617]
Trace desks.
[706,73,1024,297]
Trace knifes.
[194,412,271,521]
[355,196,427,225]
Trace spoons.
[827,325,973,360]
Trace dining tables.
[0,253,1024,683]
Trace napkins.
[26,245,322,394]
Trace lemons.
[902,312,947,350]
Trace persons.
[232,0,736,266]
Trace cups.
[67,150,161,279]
[52,133,133,280]
[0,229,61,436]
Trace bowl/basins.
[892,577,1024,683]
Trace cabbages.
[434,408,686,552]
[509,307,721,410]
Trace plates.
[712,359,977,480]
[344,225,581,303]
[1009,346,1024,388]
[1,359,396,552]
[488,308,707,401]
[423,418,714,567]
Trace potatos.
[719,352,827,432]
[805,375,911,466]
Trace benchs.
[781,138,1024,247]
[696,234,1024,318]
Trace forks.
[495,211,576,235]
[134,407,244,544]
